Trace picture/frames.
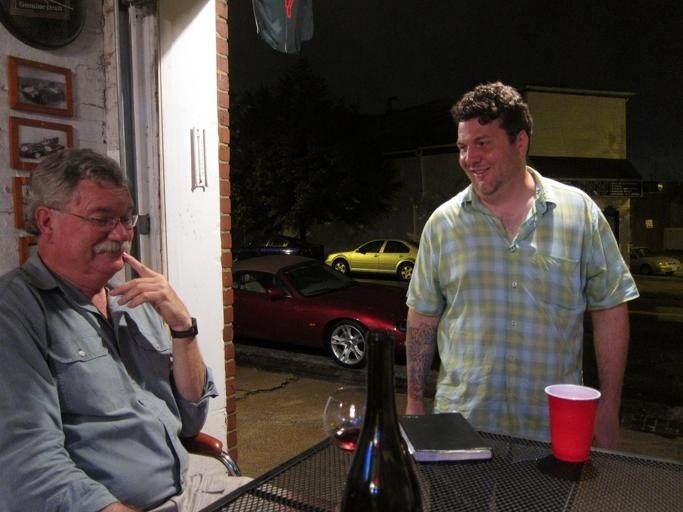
[7,110,77,175]
[8,52,79,119]
[11,171,42,233]
[18,235,44,266]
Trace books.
[397,412,493,462]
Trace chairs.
[179,427,243,477]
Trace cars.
[237,235,319,279]
[230,252,408,369]
[325,237,419,281]
[627,245,681,279]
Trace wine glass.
[320,386,375,512]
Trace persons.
[406,82,643,512]
[0,145,336,511]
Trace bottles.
[543,381,601,465]
[336,327,421,512]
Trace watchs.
[170,318,199,338]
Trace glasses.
[47,207,138,232]
